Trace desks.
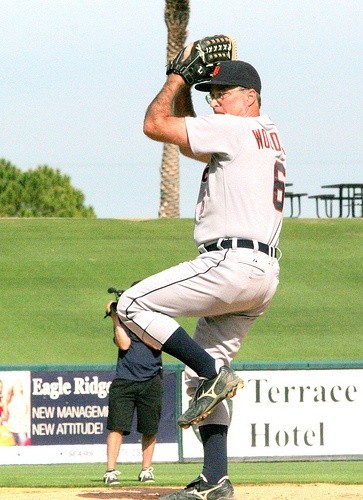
[321,184,363,218]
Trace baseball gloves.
[166,36,238,86]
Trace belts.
[205,239,276,258]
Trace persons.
[0,381,30,447]
[104,280,162,486]
[117,34,285,500]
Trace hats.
[195,60,261,94]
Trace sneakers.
[138,467,155,483]
[177,359,245,429]
[104,469,122,488]
[158,473,235,500]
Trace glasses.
[205,88,246,104]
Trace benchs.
[286,192,308,217]
[308,194,334,217]
